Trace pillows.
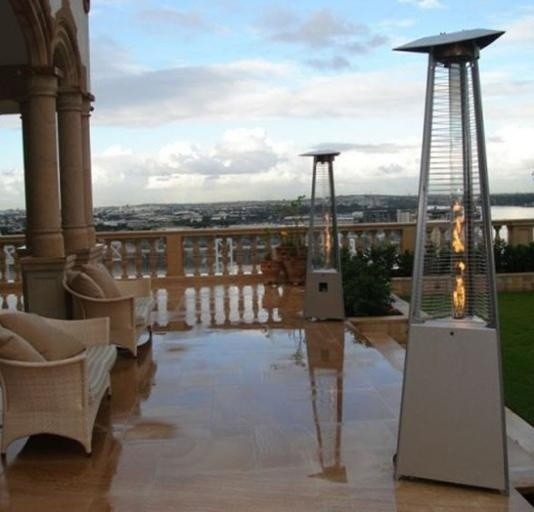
[0,326,46,362]
[82,261,122,297]
[0,309,85,360]
[67,271,105,299]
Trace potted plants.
[260,195,308,285]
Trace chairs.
[0,316,112,455]
[62,273,154,357]
